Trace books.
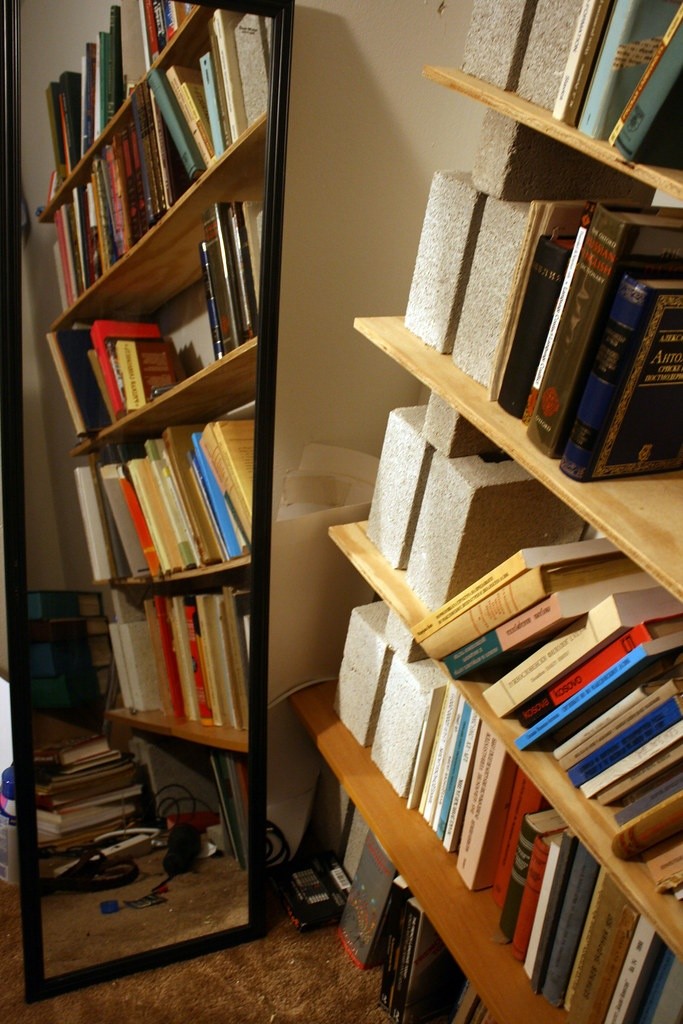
[337,0,683,1023]
[26,0,274,879]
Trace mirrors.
[2,2,292,1001]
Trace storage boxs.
[28,591,105,726]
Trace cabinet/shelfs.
[34,1,266,754]
[290,65,683,1024]
